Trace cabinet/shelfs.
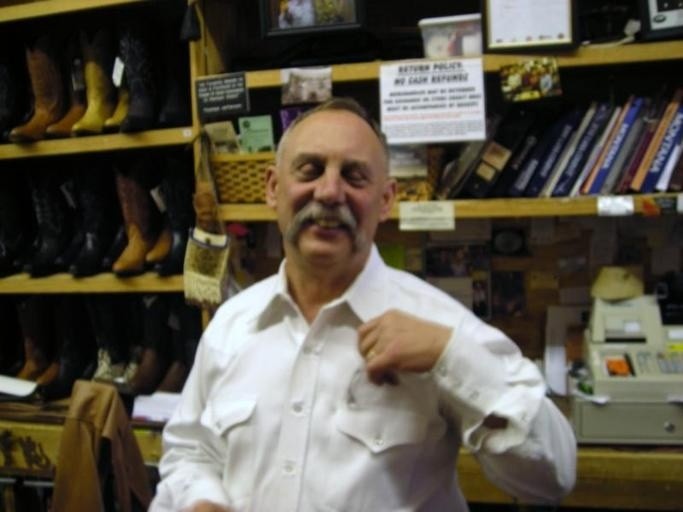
[0,0,682,512]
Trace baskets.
[209,147,444,203]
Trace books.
[442,89,682,202]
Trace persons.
[142,96,578,512]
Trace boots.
[0,155,198,278]
[0,22,193,140]
[0,293,203,399]
